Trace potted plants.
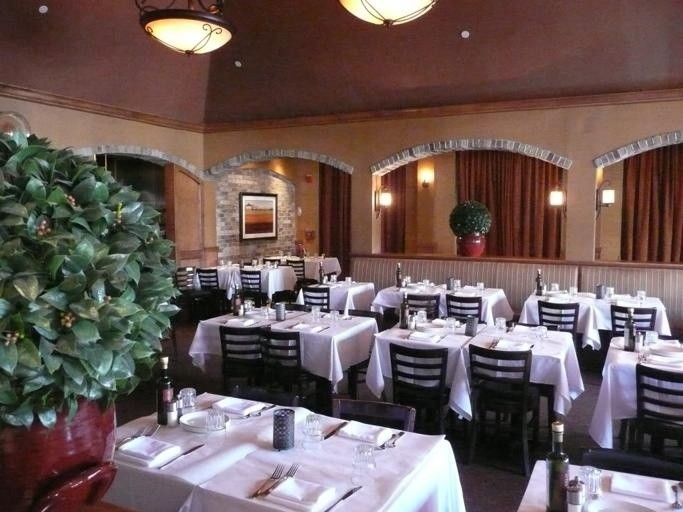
[0,129,183,430]
[450,200,492,256]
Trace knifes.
[324,485,365,512]
[159,444,205,470]
[285,323,298,329]
[323,421,348,439]
[317,326,330,334]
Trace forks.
[115,424,163,450]
[260,461,300,499]
[670,480,682,509]
[487,336,499,349]
[232,404,276,421]
[250,463,286,498]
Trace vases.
[0,394,118,512]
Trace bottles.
[399,290,409,329]
[545,421,570,512]
[166,399,176,426]
[232,283,241,316]
[153,355,174,425]
[535,268,544,297]
[567,477,585,505]
[408,312,415,330]
[317,262,324,285]
[635,332,643,350]
[395,263,402,287]
[623,307,636,352]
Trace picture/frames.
[239,192,277,242]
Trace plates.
[178,411,230,431]
[588,497,659,512]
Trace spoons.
[378,430,404,451]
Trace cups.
[551,282,646,304]
[646,331,658,342]
[179,387,197,410]
[583,466,602,492]
[243,298,339,326]
[417,310,549,343]
[216,257,279,270]
[205,407,224,431]
[324,274,352,286]
[349,444,377,486]
[404,275,485,292]
[301,414,321,448]
[278,249,325,259]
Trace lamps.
[550,184,567,218]
[596,180,615,219]
[375,184,392,219]
[418,162,434,188]
[135,0,236,56]
[339,0,441,30]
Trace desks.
[174,257,376,315]
[186,306,380,415]
[103,391,467,512]
[513,457,683,512]
[519,288,673,339]
[372,281,514,325]
[365,315,584,476]
[586,334,682,454]
